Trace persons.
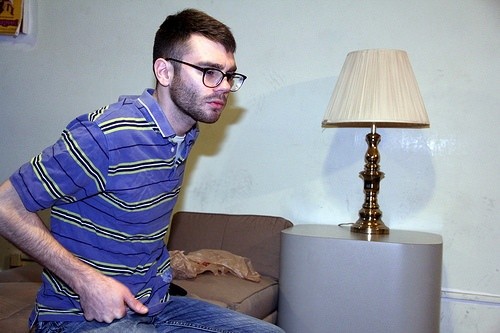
[0,8,286,332]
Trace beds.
[0,210,294,333]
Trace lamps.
[321,48,431,237]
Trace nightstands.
[276,224,443,333]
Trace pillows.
[167,212,293,281]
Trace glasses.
[164,58,247,92]
